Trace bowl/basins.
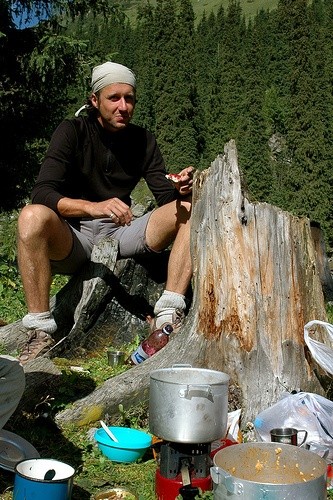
[98,426,153,464]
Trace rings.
[110,213,114,218]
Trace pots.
[150,364,230,444]
[210,441,333,500]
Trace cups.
[13,457,76,500]
[107,350,125,367]
[272,427,307,447]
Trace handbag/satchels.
[253,391,333,465]
[304,320,333,376]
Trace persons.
[17,61,196,366]
[0,355,26,431]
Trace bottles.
[124,325,173,367]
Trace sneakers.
[149,307,186,343]
[16,325,56,366]
